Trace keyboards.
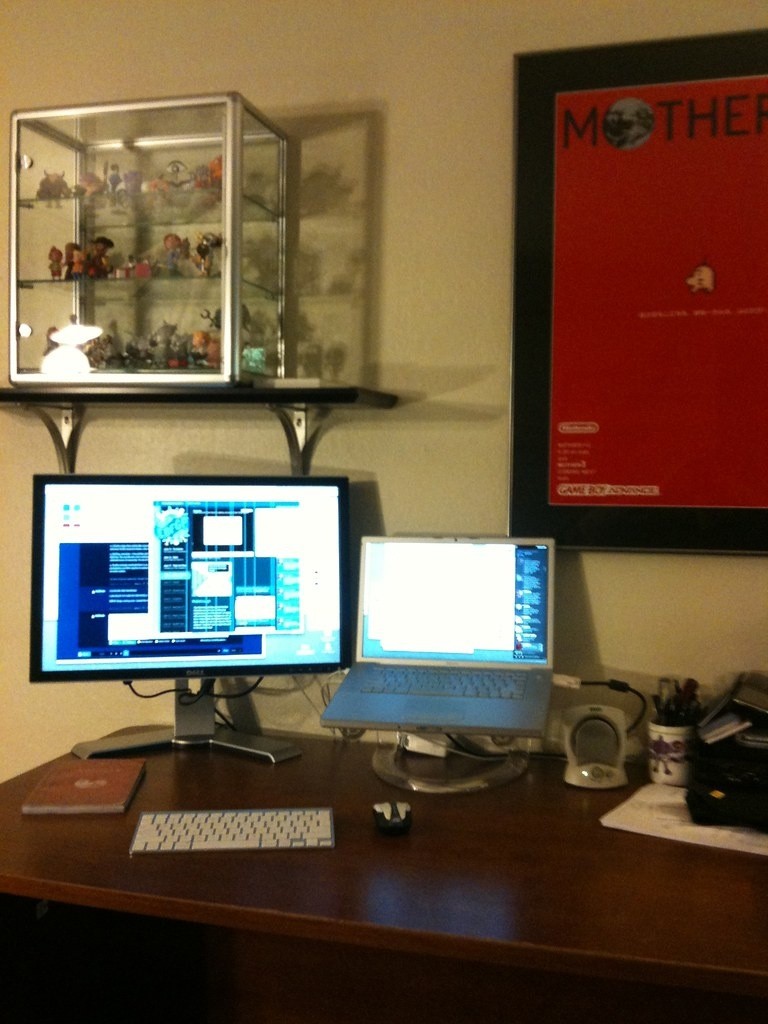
[127,807,335,854]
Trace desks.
[0,722,768,1024]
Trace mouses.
[372,800,414,836]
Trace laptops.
[319,536,555,739]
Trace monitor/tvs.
[31,473,355,763]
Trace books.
[21,757,148,813]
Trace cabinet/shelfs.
[8,90,293,392]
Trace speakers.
[561,704,630,788]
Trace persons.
[47,232,221,278]
